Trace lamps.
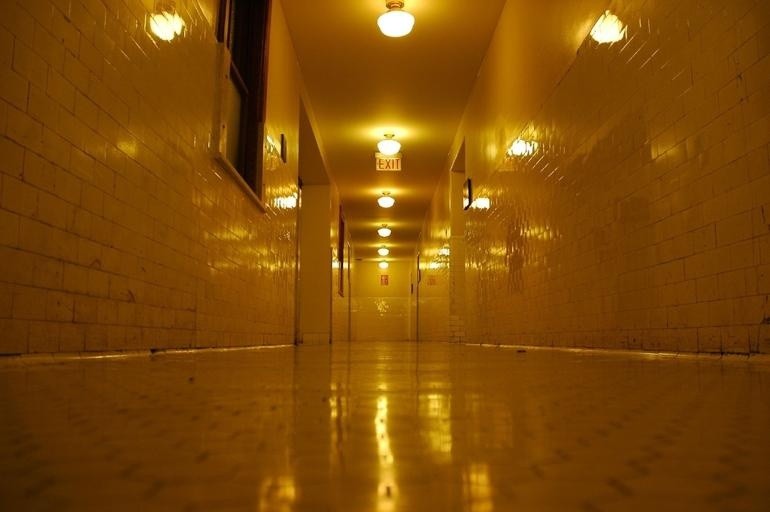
[377,0,417,38]
[376,133,403,269]
